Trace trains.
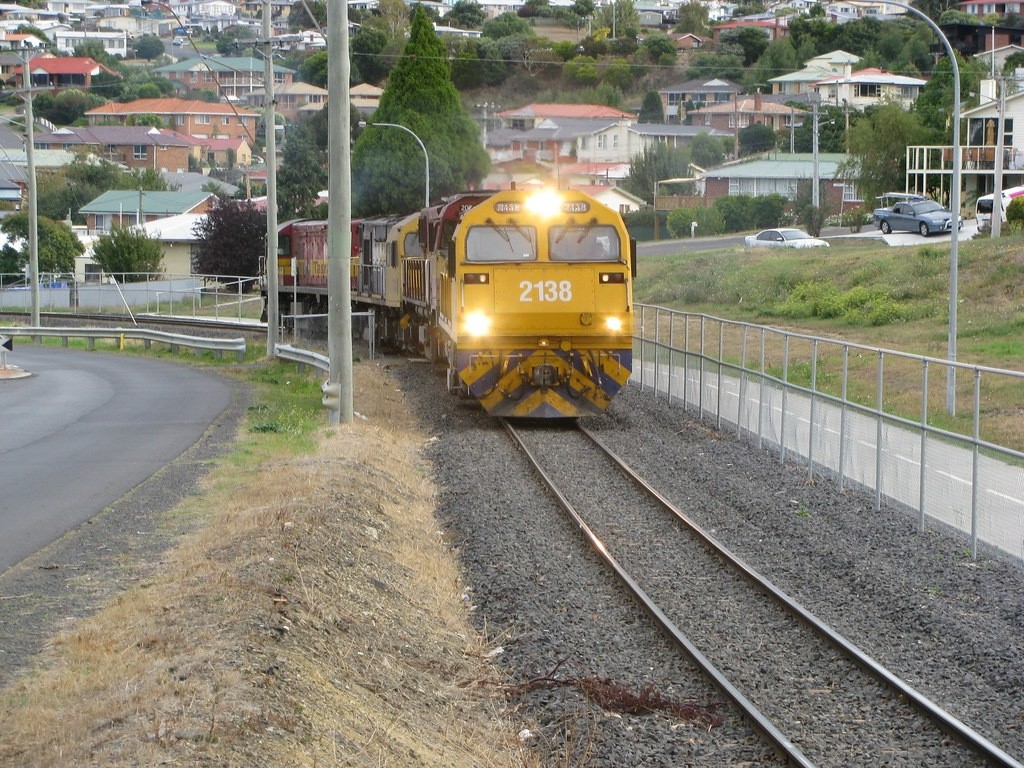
[255,182,638,422]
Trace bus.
[975,186,1024,234]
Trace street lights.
[358,120,431,209]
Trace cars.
[744,226,830,250]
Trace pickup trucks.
[874,198,964,237]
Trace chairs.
[944,148,1019,170]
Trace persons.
[584,242,608,259]
